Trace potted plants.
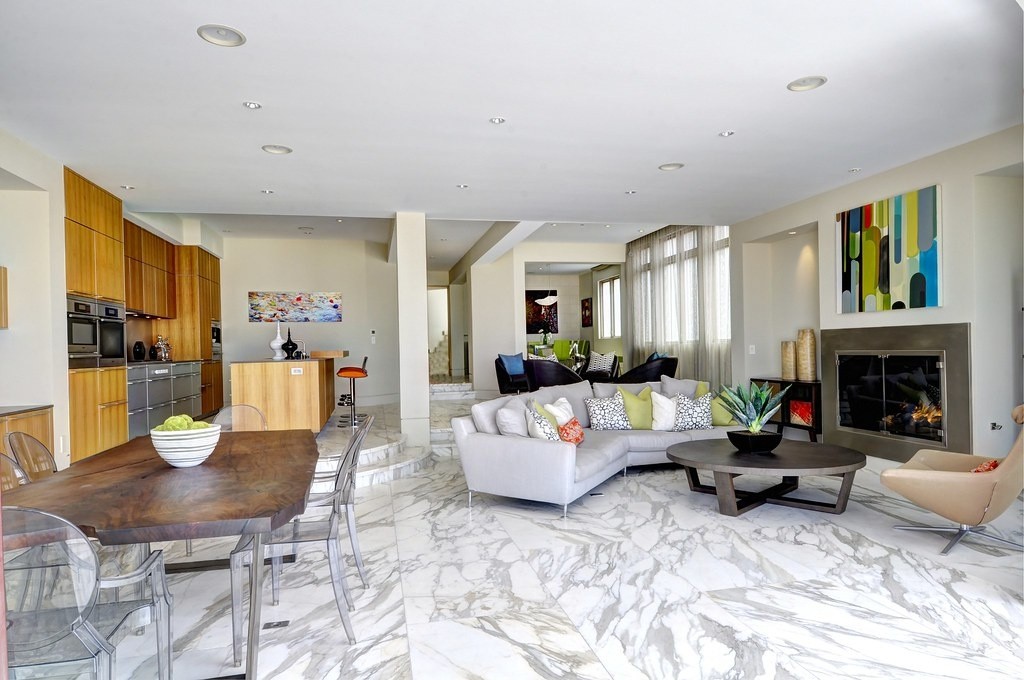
[717,379,790,452]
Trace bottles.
[149,345,157,360]
[133,341,146,360]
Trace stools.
[337,356,368,427]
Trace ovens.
[66,292,100,368]
[210,320,222,361]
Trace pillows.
[528,352,559,363]
[584,384,740,433]
[586,350,615,374]
[498,352,524,374]
[496,396,586,445]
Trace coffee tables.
[668,439,865,518]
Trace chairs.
[577,354,619,383]
[616,358,679,384]
[525,358,583,393]
[882,403,1023,554]
[493,353,528,395]
[554,340,571,360]
[0,416,378,680]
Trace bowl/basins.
[150,424,221,468]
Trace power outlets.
[372,337,376,344]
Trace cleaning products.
[542,333,547,345]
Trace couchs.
[451,375,746,520]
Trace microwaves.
[97,299,126,367]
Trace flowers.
[538,329,552,340]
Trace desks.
[0,429,320,680]
[534,342,555,356]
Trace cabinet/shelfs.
[153,244,224,418]
[127,362,203,440]
[123,216,177,319]
[63,166,127,466]
[751,377,821,443]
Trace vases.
[543,336,547,345]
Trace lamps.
[535,264,559,306]
